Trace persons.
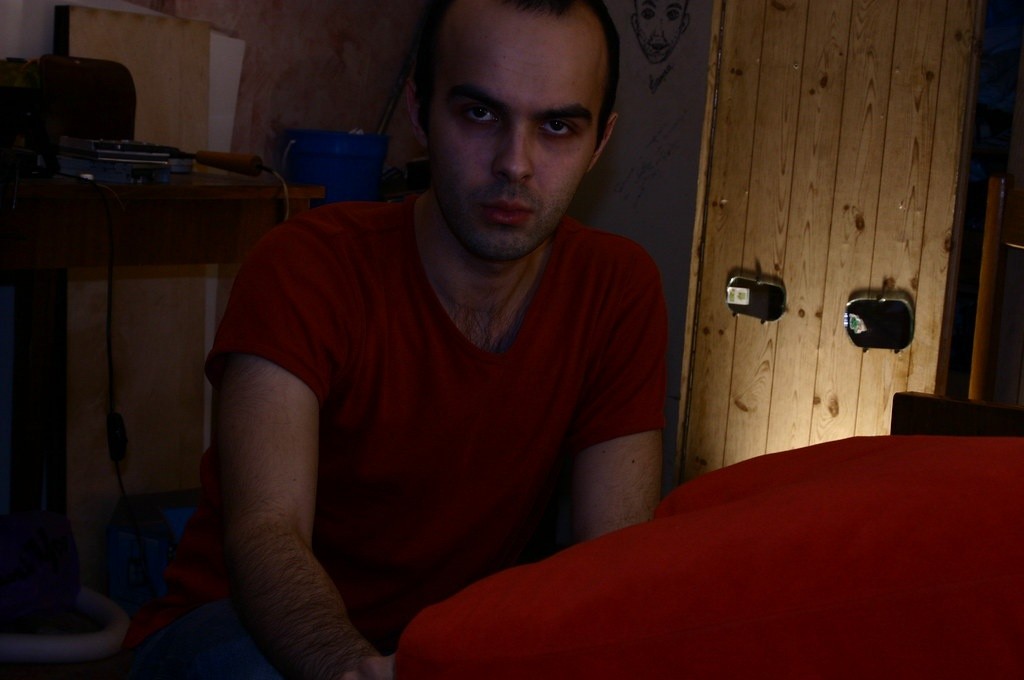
[123,0,667,680]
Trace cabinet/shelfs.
[0,166,326,603]
